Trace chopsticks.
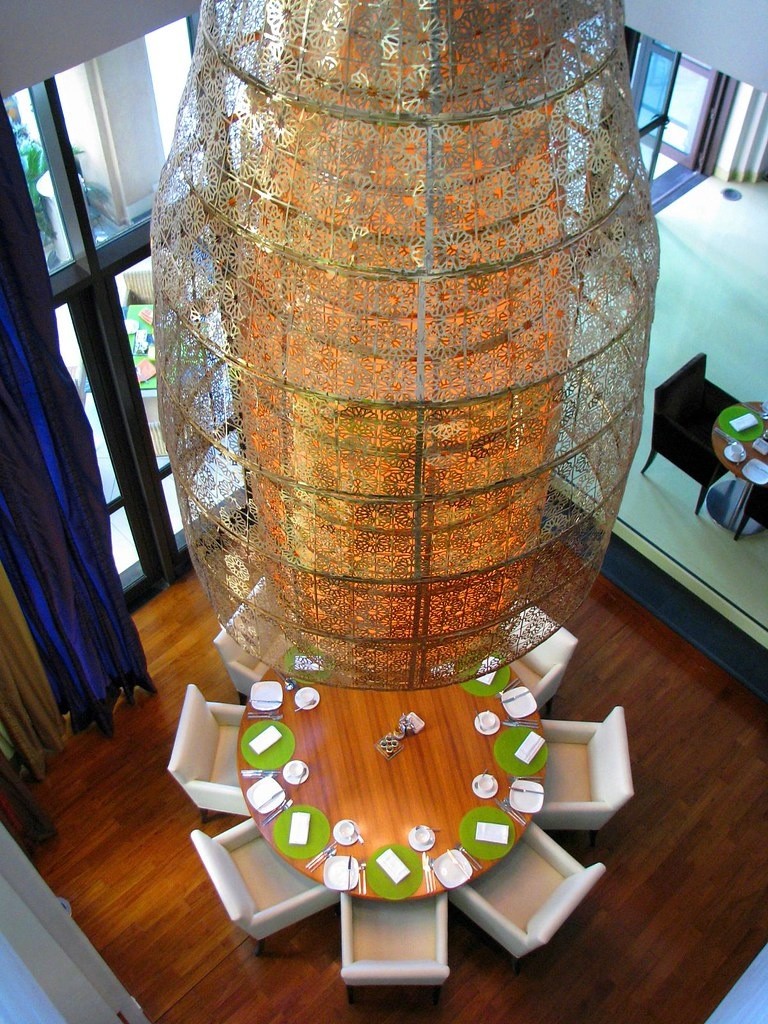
[508,810,526,826]
[306,841,336,869]
[424,853,433,893]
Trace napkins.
[376,849,411,884]
[289,812,311,844]
[476,656,499,686]
[430,662,456,677]
[294,656,325,672]
[730,414,758,431]
[249,726,283,755]
[475,822,510,844]
[514,732,545,764]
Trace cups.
[415,829,433,845]
[287,764,304,778]
[478,775,494,792]
[340,823,354,842]
[480,709,495,728]
[731,445,742,457]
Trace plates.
[501,686,537,718]
[472,774,498,799]
[433,849,473,888]
[324,856,359,890]
[724,446,746,462]
[283,760,309,785]
[333,820,360,846]
[408,825,435,851]
[295,687,320,710]
[510,780,544,813]
[742,459,768,485]
[247,777,285,814]
[251,681,283,711]
[475,712,500,735]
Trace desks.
[124,305,158,390]
[712,401,768,536]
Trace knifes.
[502,691,531,702]
[348,856,351,890]
[447,849,470,880]
[509,787,543,794]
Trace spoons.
[263,799,293,824]
[475,769,487,788]
[360,860,366,894]
[296,768,306,790]
[349,822,364,844]
[455,842,483,869]
[310,849,336,872]
[495,677,518,698]
[274,669,294,690]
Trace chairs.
[641,353,741,514]
[166,683,254,823]
[448,821,606,976]
[121,271,155,306]
[733,484,768,540]
[190,818,340,956]
[531,706,634,846]
[338,892,450,1007]
[214,575,578,713]
[143,397,169,456]
